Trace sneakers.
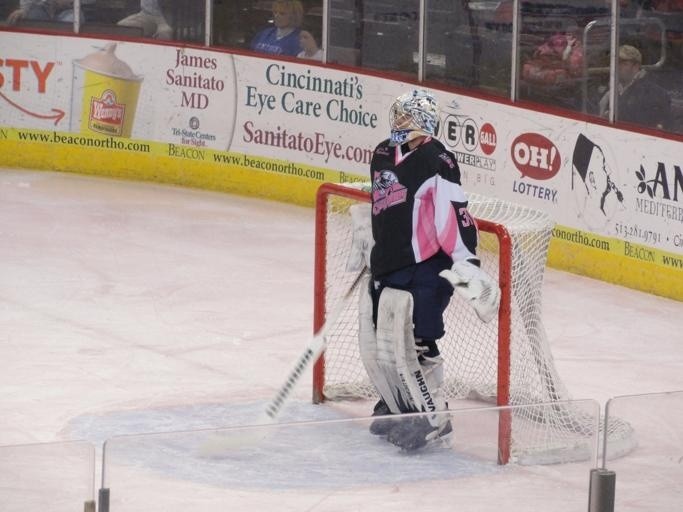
[370,414,451,445]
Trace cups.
[68,61,143,138]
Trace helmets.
[388,89,439,145]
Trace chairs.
[0,0,682,142]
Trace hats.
[618,44,640,61]
[298,21,322,47]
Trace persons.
[358,90,502,454]
[0,0,683,133]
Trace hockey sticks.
[214,266,365,454]
